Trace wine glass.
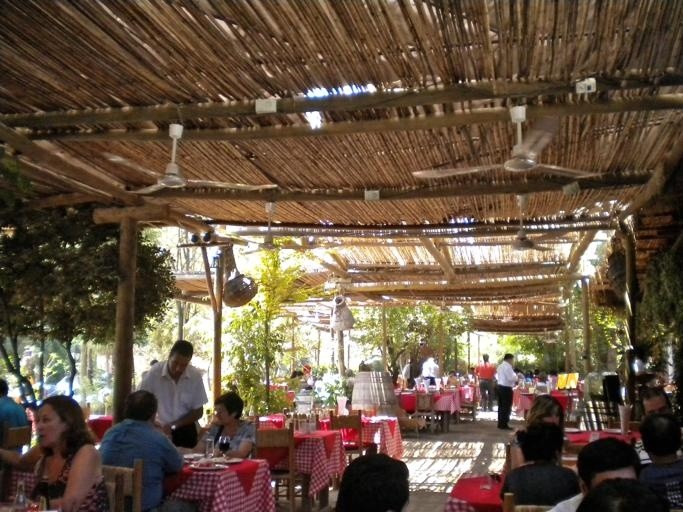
[218,435,231,457]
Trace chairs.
[503,492,553,512]
[415,392,443,434]
[102,458,143,512]
[275,413,320,504]
[460,386,477,423]
[2,420,33,451]
[349,408,376,417]
[252,422,314,512]
[330,409,363,489]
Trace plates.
[183,453,243,470]
[602,428,633,434]
[563,428,580,433]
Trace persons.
[0,354,565,430]
[0,378,29,469]
[502,387,682,512]
[332,455,408,512]
[0,395,109,512]
[138,340,207,448]
[99,390,198,512]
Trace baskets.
[222,274,258,307]
[331,295,354,332]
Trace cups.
[589,433,600,443]
[206,438,214,458]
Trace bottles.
[205,409,212,425]
[14,484,26,512]
[239,406,254,420]
[284,412,317,435]
[39,475,50,512]
